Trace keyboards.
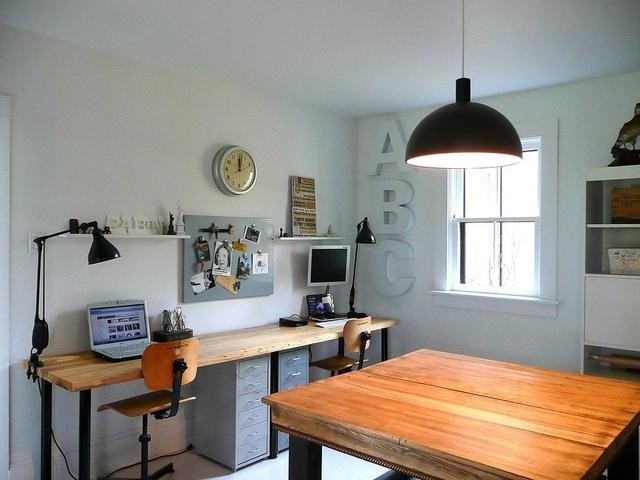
[315,318,354,328]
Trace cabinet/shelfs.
[191,353,270,475]
[272,347,311,453]
[579,162,639,378]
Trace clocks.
[211,143,258,197]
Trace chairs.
[307,314,373,379]
[95,335,201,478]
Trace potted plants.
[151,306,193,343]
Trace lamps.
[24,220,123,383]
[402,1,526,171]
[344,216,377,318]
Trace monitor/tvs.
[307,244,351,284]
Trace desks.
[23,312,400,480]
[256,344,639,479]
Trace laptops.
[88,298,152,360]
[306,294,347,320]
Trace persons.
[213,244,231,275]
[237,252,250,280]
